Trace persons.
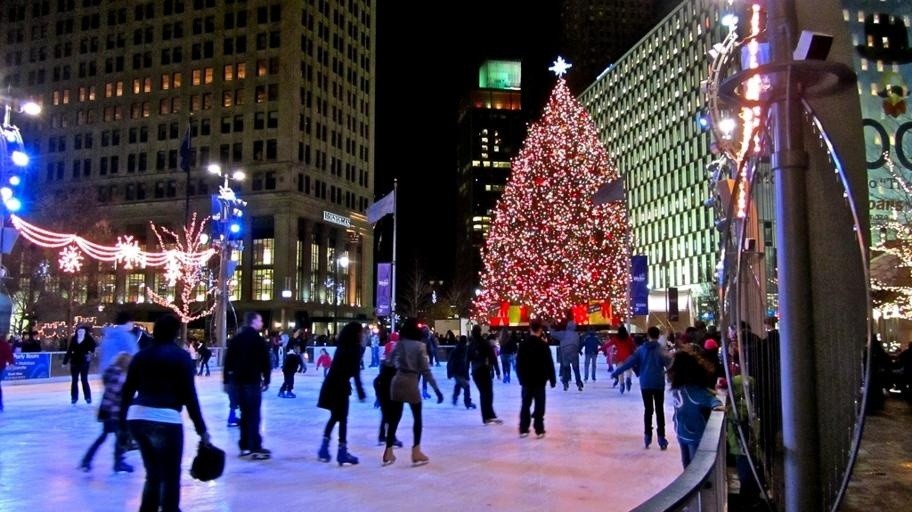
[116,314,212,510]
[63,311,154,478]
[189,310,727,470]
[727,320,780,470]
[869,333,911,416]
[1,331,69,411]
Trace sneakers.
[114,462,134,473]
[278,392,296,398]
[620,378,631,392]
[645,435,651,444]
[658,437,668,445]
[239,440,271,453]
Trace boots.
[383,446,395,462]
[228,409,240,423]
[337,443,358,462]
[412,445,429,463]
[319,439,331,460]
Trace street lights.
[0,85,44,131]
[207,163,247,350]
[429,280,444,319]
[332,248,349,335]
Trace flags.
[365,190,393,225]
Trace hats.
[704,338,719,350]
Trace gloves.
[435,389,443,404]
[357,388,365,400]
